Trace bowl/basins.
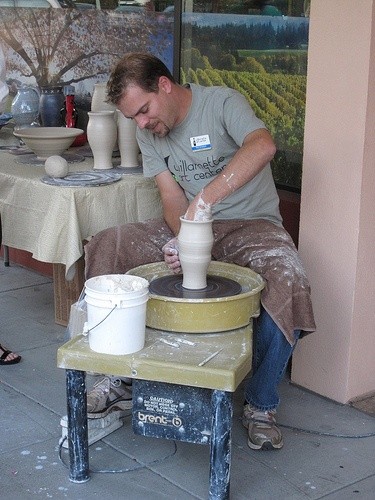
[12,127,84,160]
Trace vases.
[10,86,40,129]
[38,84,65,126]
[88,109,116,171]
[116,109,139,168]
[175,213,215,291]
[90,83,114,112]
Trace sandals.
[0,344,21,364]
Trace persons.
[86,53,317,449]
[0,343,21,365]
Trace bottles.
[10,88,39,127]
[39,86,67,126]
[91,83,118,151]
[63,84,76,127]
[177,215,214,290]
[86,111,116,169]
[116,109,140,167]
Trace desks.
[50,319,254,500]
[0,125,164,332]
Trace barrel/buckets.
[82,274,149,355]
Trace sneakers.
[86,377,133,418]
[242,403,284,450]
